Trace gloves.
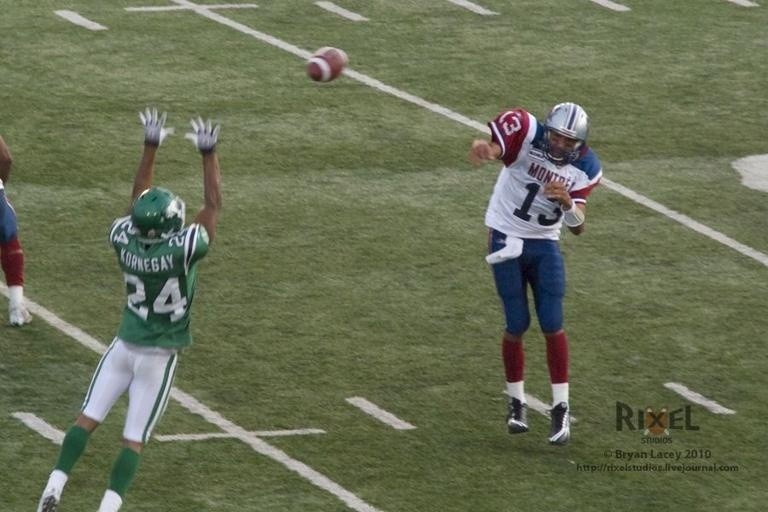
[184,115,220,152]
[138,106,176,144]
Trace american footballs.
[306,46,349,83]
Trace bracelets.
[557,199,585,230]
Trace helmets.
[131,186,186,239]
[538,102,591,166]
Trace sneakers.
[8,300,33,327]
[37,487,60,512]
[506,397,529,434]
[546,402,570,444]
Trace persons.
[0,132,34,327]
[36,104,227,512]
[470,103,606,447]
